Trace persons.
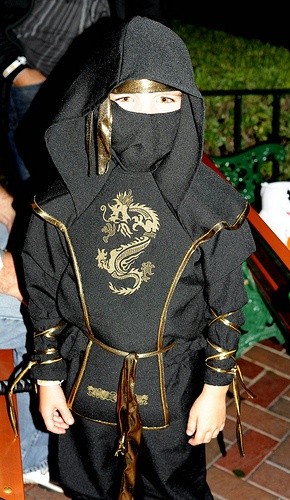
[11,16,259,500]
[0,0,113,493]
